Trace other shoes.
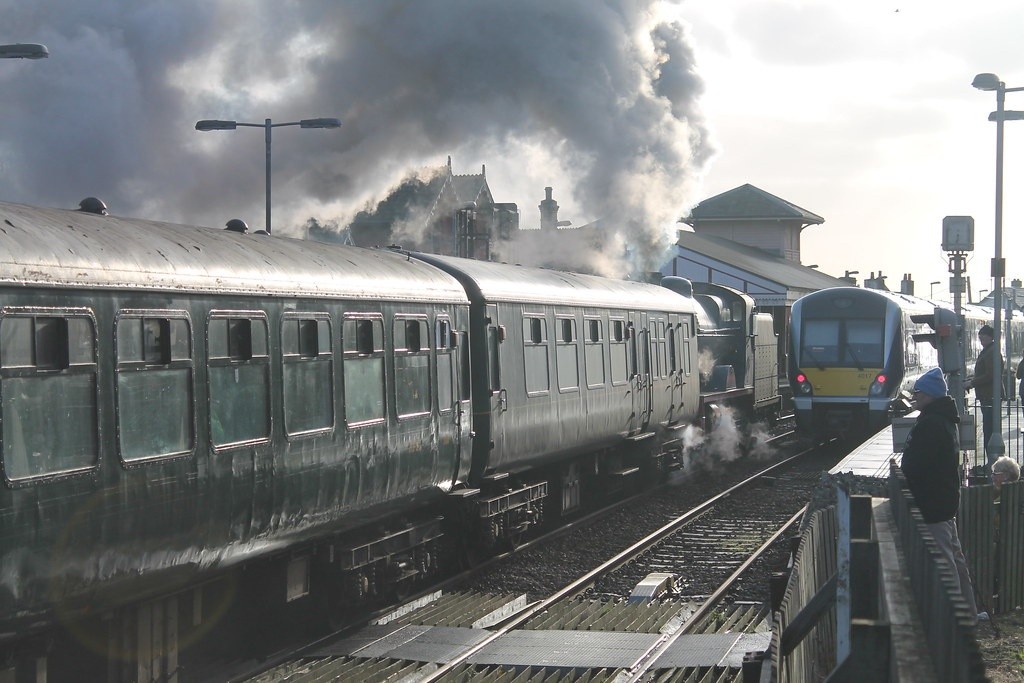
[972,462,991,475]
[1021,427,1024,433]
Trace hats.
[914,367,947,398]
[978,325,994,340]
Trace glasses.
[991,472,1004,477]
[913,389,921,395]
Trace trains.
[0,201,785,683]
[785,286,1023,448]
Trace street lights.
[195,115,341,235]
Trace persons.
[1016,349,1024,418]
[900,367,981,629]
[961,324,1006,467]
